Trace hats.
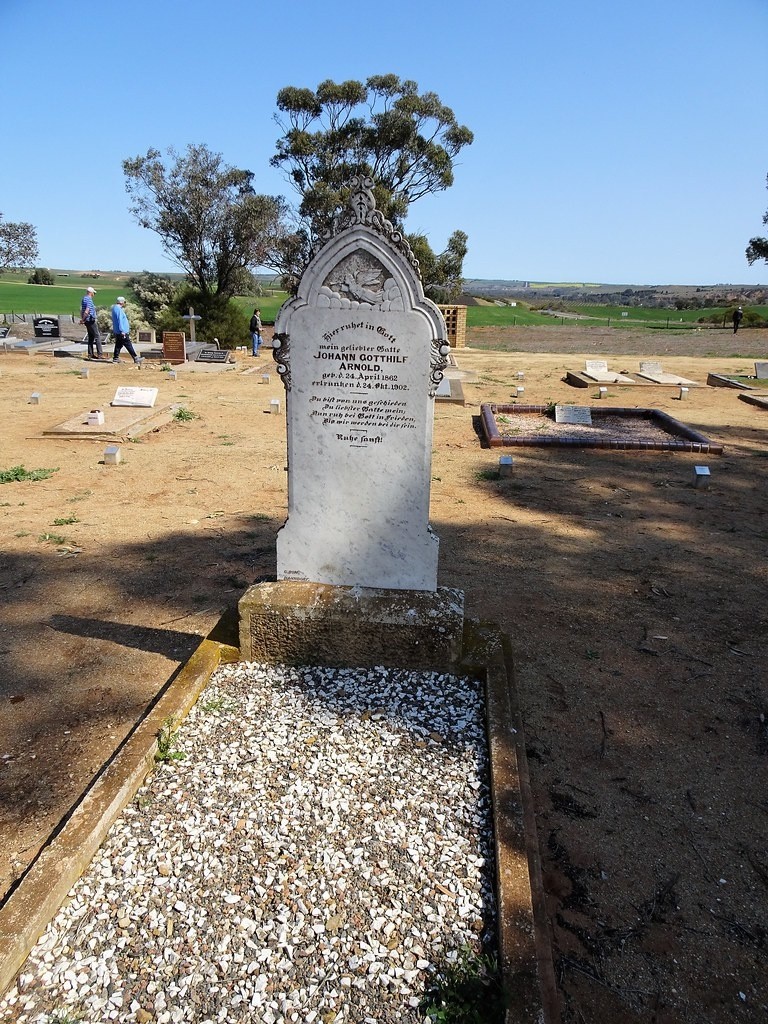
[86,287,97,293]
[117,297,125,303]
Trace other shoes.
[88,354,97,359]
[253,353,261,357]
[98,356,107,359]
[113,358,125,364]
[134,356,145,363]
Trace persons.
[249,308,263,358]
[79,287,106,359]
[732,306,742,333]
[111,297,145,364]
